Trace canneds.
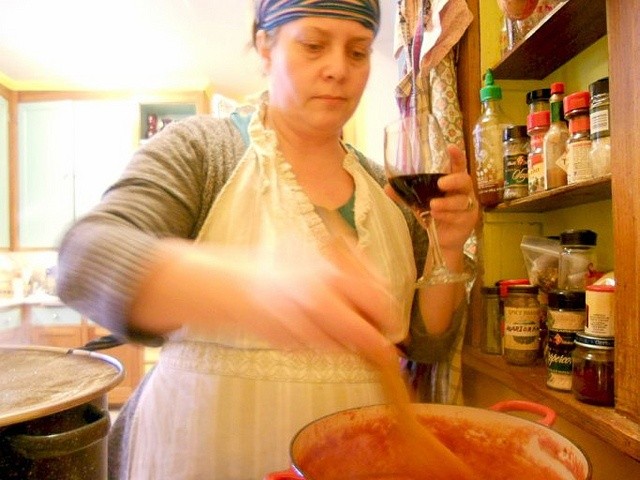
[483,286,502,355]
[572,331,614,408]
[547,289,584,392]
[497,279,530,335]
[502,284,541,366]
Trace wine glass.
[384,113,474,290]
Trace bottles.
[543,82,570,190]
[474,69,515,207]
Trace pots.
[262,399,593,479]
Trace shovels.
[315,218,473,480]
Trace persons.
[57,2,480,479]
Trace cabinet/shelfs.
[473,0,640,461]
[38,325,141,404]
